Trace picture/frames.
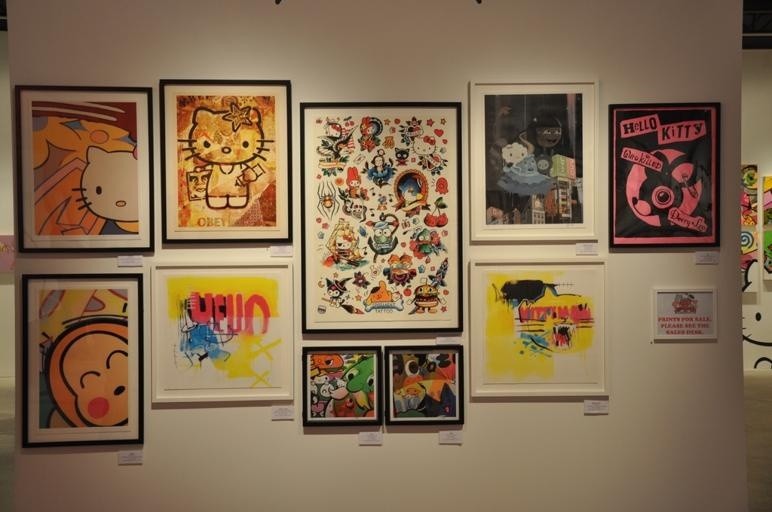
[385,344,464,425]
[300,101,464,333]
[15,84,155,254]
[470,79,599,241]
[158,78,293,244]
[467,256,610,398]
[608,102,721,248]
[650,288,718,341]
[21,272,144,448]
[145,260,294,403]
[302,345,383,427]
